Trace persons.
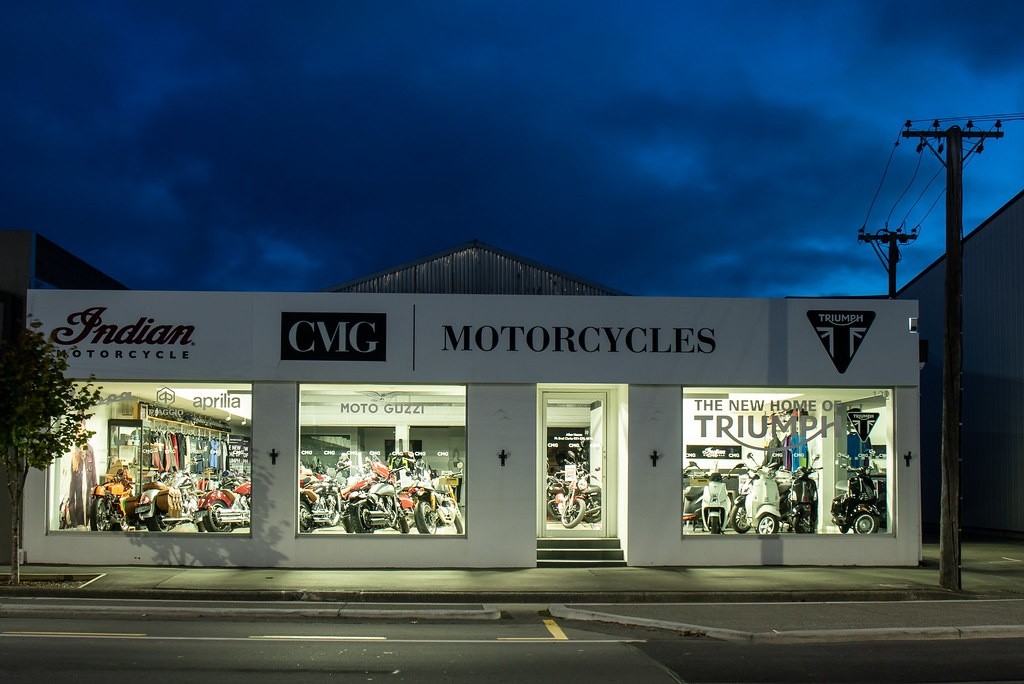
[68,410,97,529]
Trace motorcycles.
[89,467,250,532]
[546,451,602,528]
[300,454,464,533]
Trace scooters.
[683,453,824,534]
[831,464,881,534]
